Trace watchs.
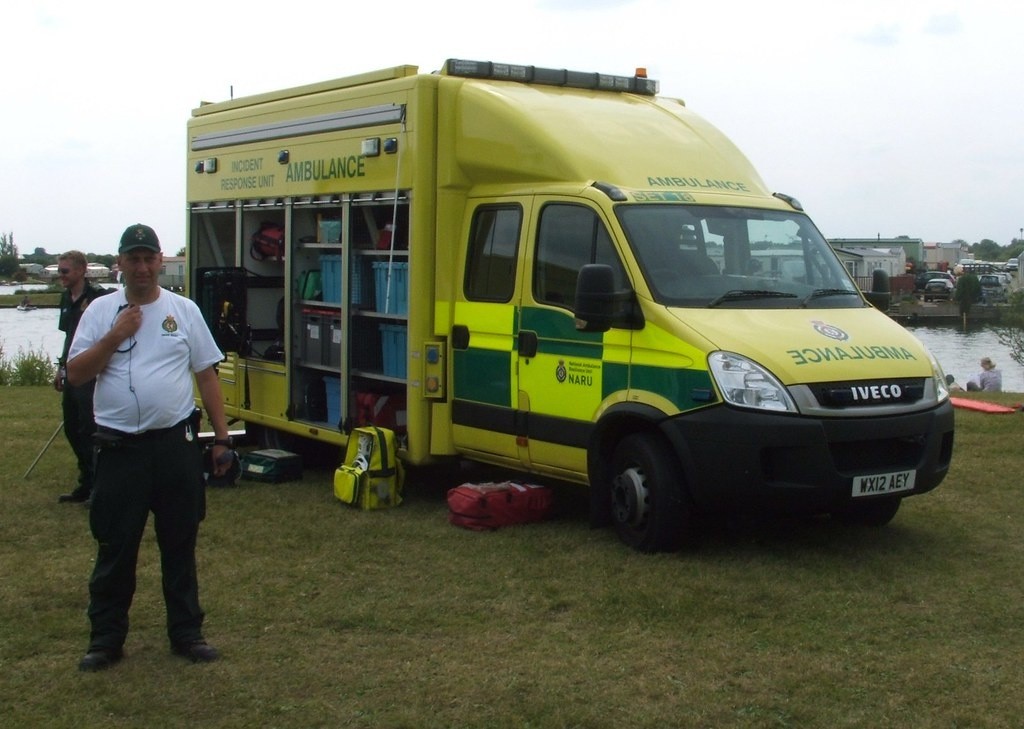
[214,436,233,445]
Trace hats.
[119,224,161,254]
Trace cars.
[924,278,953,302]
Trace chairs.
[657,249,724,280]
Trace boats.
[18,305,37,310]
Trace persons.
[67,224,233,671]
[946,374,965,392]
[967,357,1002,391]
[22,296,31,307]
[54,250,107,504]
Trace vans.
[1007,258,1020,273]
[920,271,956,288]
[978,274,1009,300]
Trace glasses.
[58,268,69,274]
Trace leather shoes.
[172,638,219,661]
[80,643,124,671]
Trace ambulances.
[179,57,956,553]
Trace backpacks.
[251,219,285,266]
[448,483,566,529]
[333,424,406,510]
[304,379,327,422]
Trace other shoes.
[60,483,92,503]
[86,490,96,508]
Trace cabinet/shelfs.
[185,188,410,459]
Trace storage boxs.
[322,376,342,426]
[320,221,342,243]
[242,448,305,483]
[379,324,407,380]
[372,262,408,317]
[318,255,342,303]
